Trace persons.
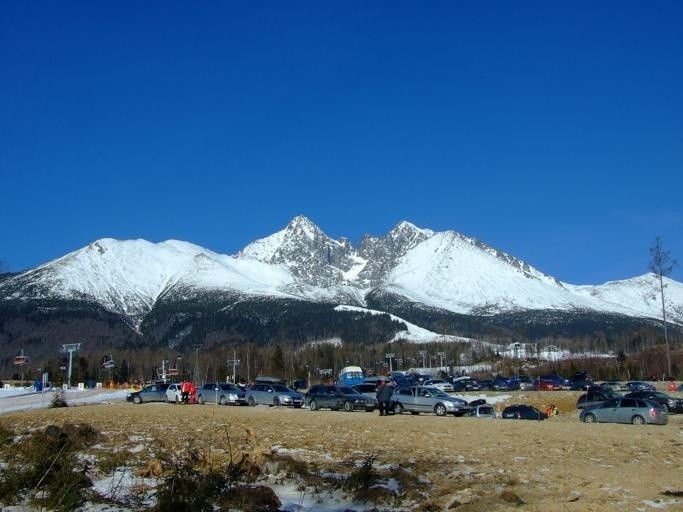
[667,378,676,397]
[179,379,192,405]
[375,380,391,417]
[237,377,253,391]
